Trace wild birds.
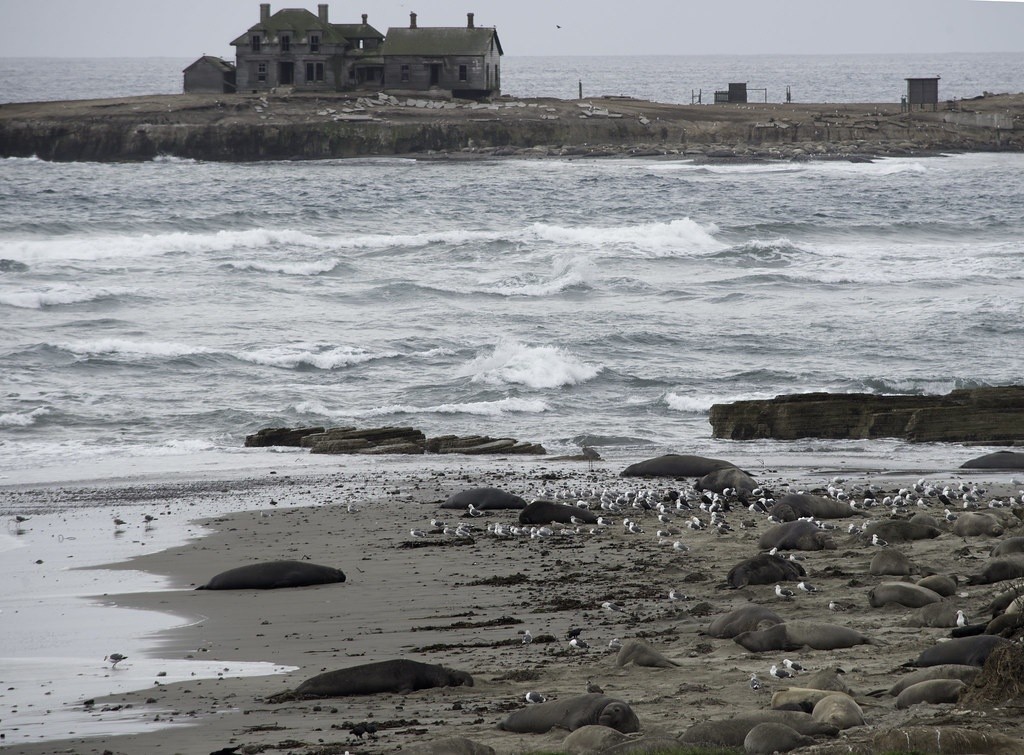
[7,484,1023,707]
